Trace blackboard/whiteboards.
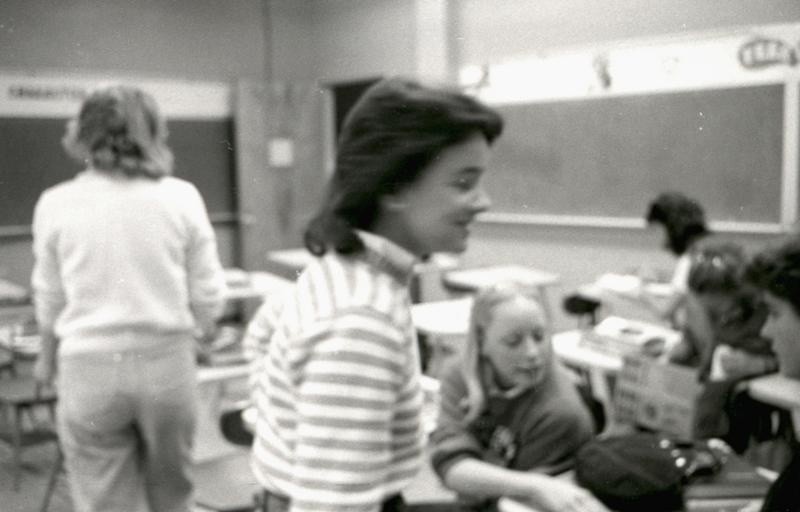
[470,77,799,236]
[0,113,236,237]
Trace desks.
[0,248,798,512]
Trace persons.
[241,76,503,512]
[667,237,775,453]
[428,281,610,512]
[738,244,799,512]
[642,185,715,374]
[25,80,230,511]
